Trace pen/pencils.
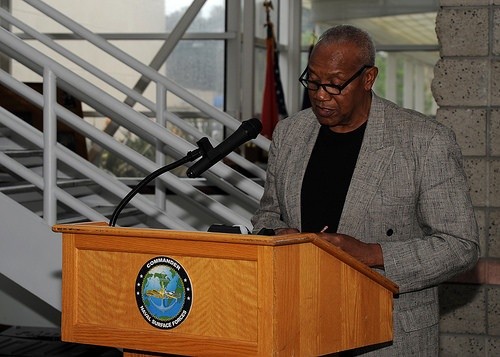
[318,224,329,234]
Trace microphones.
[186,118,263,178]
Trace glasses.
[299,64,374,95]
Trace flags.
[260,23,289,141]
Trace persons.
[251,25,480,357]
